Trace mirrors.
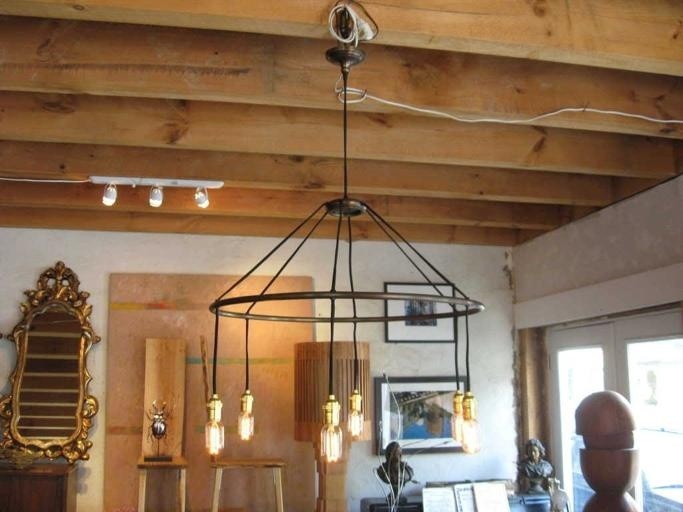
[0,258,103,471]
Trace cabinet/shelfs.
[1,463,80,511]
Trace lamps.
[202,4,489,465]
[86,174,226,209]
[292,339,373,512]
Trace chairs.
[138,336,192,512]
[198,335,287,512]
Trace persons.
[515,438,554,496]
[375,441,412,487]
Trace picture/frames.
[373,375,472,457]
[382,280,459,343]
